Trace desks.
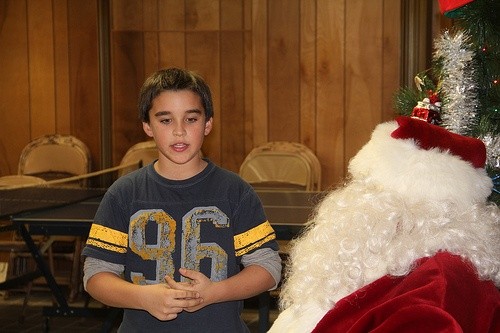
[0,185,332,333]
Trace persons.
[82,66,285,333]
[262,114,499,332]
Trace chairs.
[119,141,206,181]
[1,131,93,305]
[235,139,323,191]
